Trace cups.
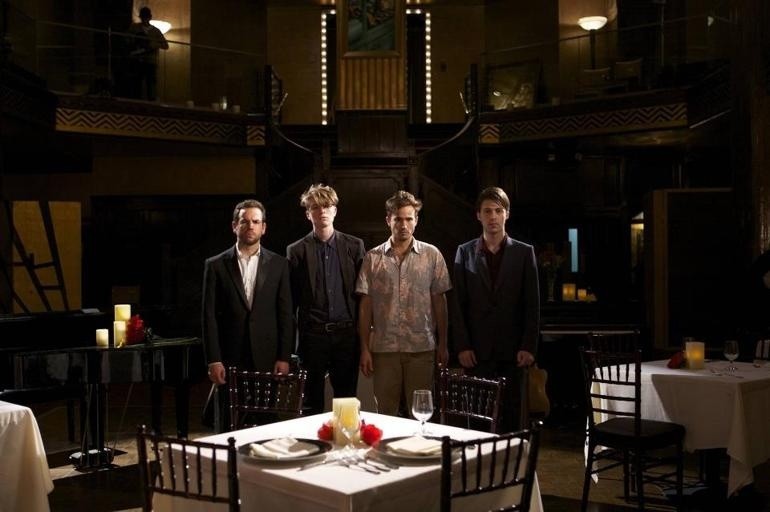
[685,341,704,369]
[563,284,586,300]
[754,340,770,367]
[95,304,131,348]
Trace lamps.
[577,289,586,300]
[150,19,173,36]
[562,283,576,301]
[577,15,608,67]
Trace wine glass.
[723,340,739,377]
[411,388,435,437]
[337,402,361,452]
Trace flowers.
[667,353,684,369]
[318,418,382,446]
[124,315,162,347]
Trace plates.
[237,436,334,462]
[370,435,464,461]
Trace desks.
[46,332,207,451]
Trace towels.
[384,433,451,455]
[247,432,318,460]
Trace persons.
[129,6,169,101]
[285,183,366,417]
[451,186,540,438]
[202,199,292,433]
[354,190,452,420]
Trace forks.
[335,449,400,475]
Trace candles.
[113,320,126,347]
[114,304,132,322]
[96,328,110,346]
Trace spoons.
[711,368,745,381]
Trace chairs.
[575,341,686,511]
[224,365,308,430]
[134,423,242,511]
[437,366,507,432]
[439,419,545,510]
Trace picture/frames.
[337,0,404,60]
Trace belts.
[305,321,352,333]
